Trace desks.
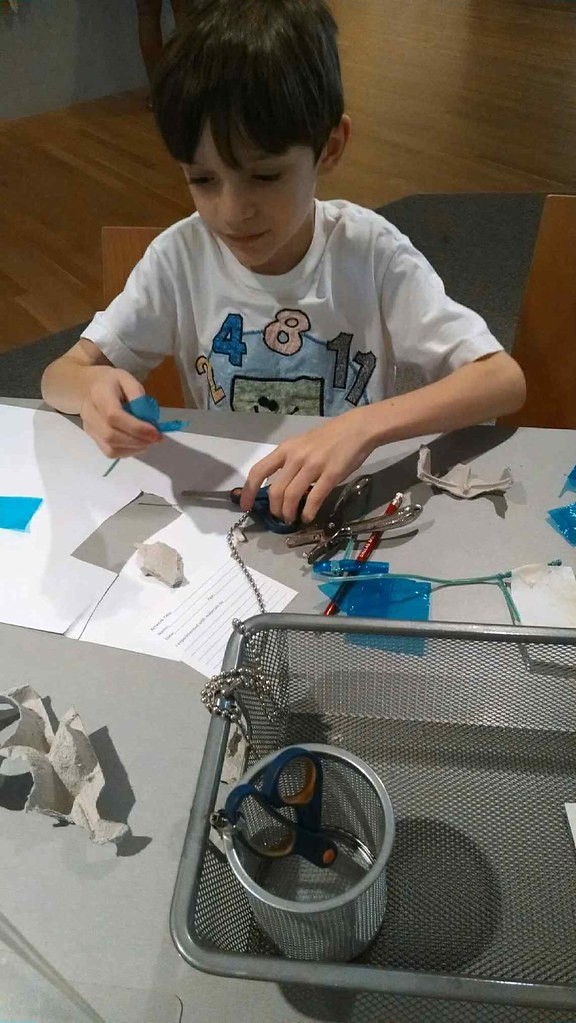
[0,391,576,1023]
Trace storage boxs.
[167,607,575,1012]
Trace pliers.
[285,475,424,562]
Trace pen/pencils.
[324,491,404,617]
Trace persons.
[36,1,535,532]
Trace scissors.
[181,481,305,533]
[224,748,368,883]
